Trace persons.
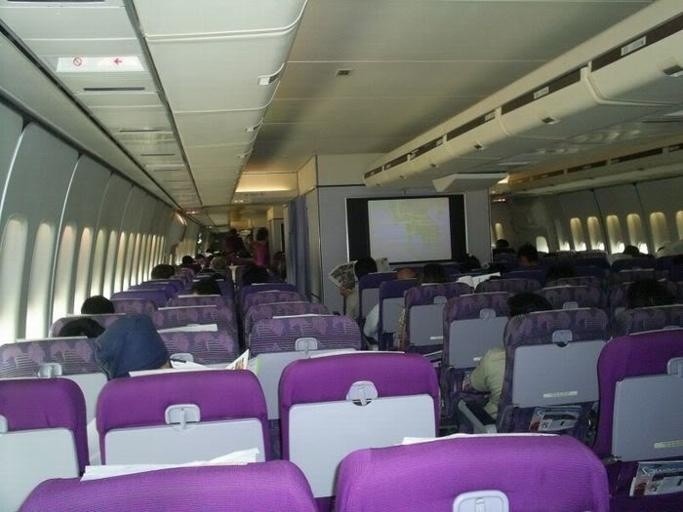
[85,312,172,464]
[82,295,115,313]
[148,226,271,296]
[463,292,554,422]
[362,268,417,351]
[397,263,450,351]
[339,257,377,319]
[418,239,683,309]
[56,318,104,339]
